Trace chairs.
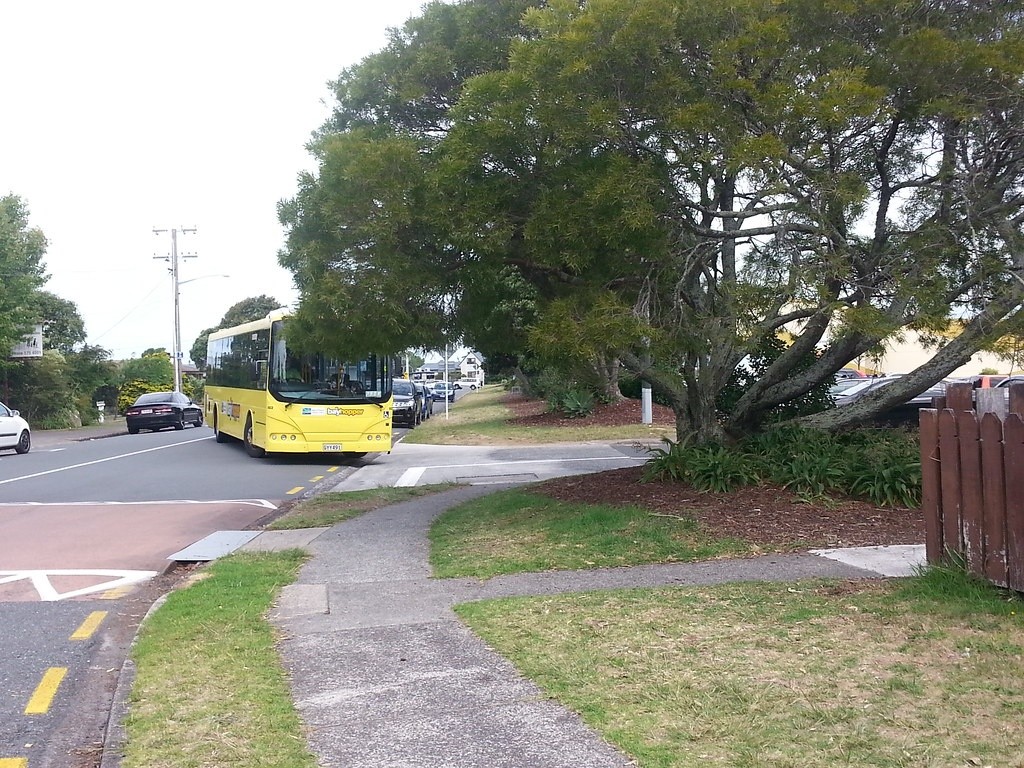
[331,373,350,386]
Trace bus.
[202,304,403,460]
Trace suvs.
[453,377,481,390]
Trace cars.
[364,376,444,429]
[125,391,203,434]
[429,381,458,402]
[820,366,1024,431]
[0,400,32,455]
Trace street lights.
[174,273,232,394]
